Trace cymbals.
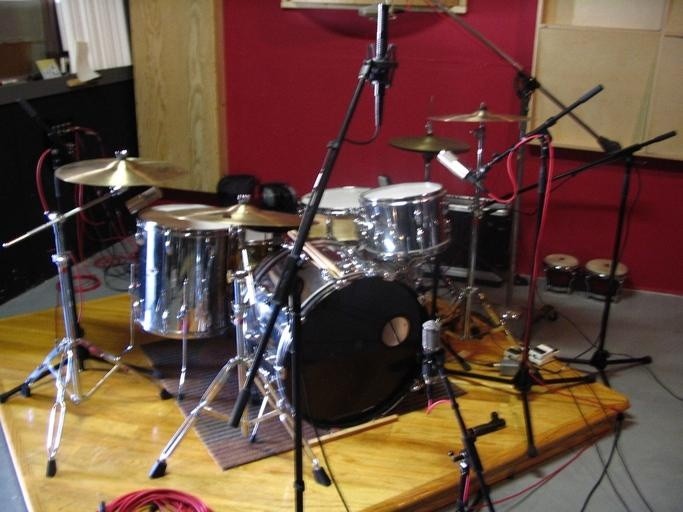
[389,136,472,154]
[429,108,532,125]
[55,154,185,188]
[192,200,298,230]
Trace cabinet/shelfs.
[0,64,139,305]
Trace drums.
[299,186,369,243]
[542,252,581,295]
[242,239,425,431]
[354,181,452,262]
[583,257,629,302]
[130,204,239,340]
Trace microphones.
[423,321,440,398]
[373,3,389,126]
[437,149,491,196]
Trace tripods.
[444,84,604,459]
[431,257,471,372]
[487,131,675,420]
[2,190,171,478]
[1,97,166,402]
[436,123,515,347]
[149,231,331,485]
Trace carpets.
[140,330,468,472]
